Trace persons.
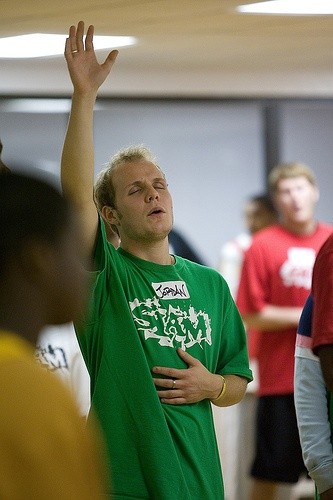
[223,195,278,499]
[0,169,105,500]
[235,162,333,500]
[293,231,333,500]
[59,21,253,500]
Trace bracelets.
[213,375,226,400]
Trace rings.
[172,379,176,389]
[72,49,79,54]
